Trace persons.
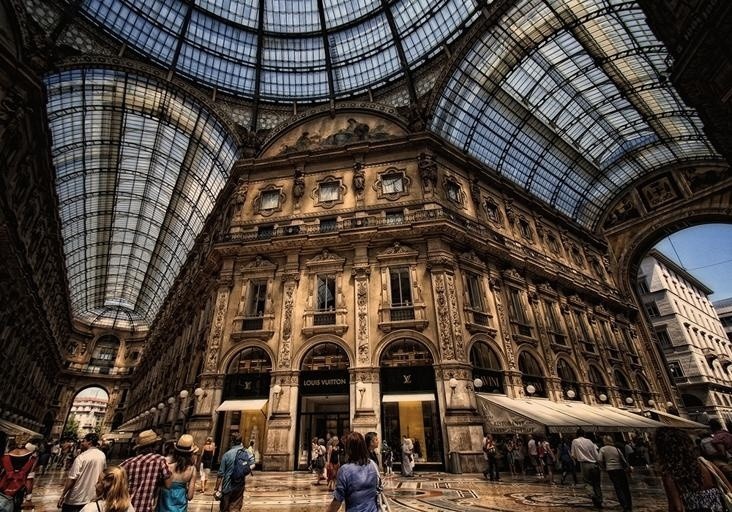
[482,420,732,512]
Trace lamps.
[620,396,634,405]
[273,384,284,399]
[465,379,484,394]
[643,399,656,408]
[519,385,537,398]
[592,394,607,404]
[1,409,47,429]
[448,377,459,396]
[663,400,673,411]
[116,386,209,430]
[356,380,366,397]
[559,389,576,402]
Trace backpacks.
[232,449,251,483]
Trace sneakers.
[482,473,578,483]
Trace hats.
[174,433,199,457]
[133,429,164,449]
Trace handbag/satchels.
[368,458,392,512]
